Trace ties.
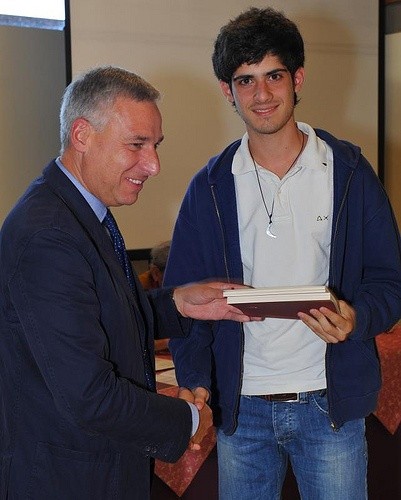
[104,214,156,392]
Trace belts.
[260,393,300,401]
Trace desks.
[150,320,401,500]
